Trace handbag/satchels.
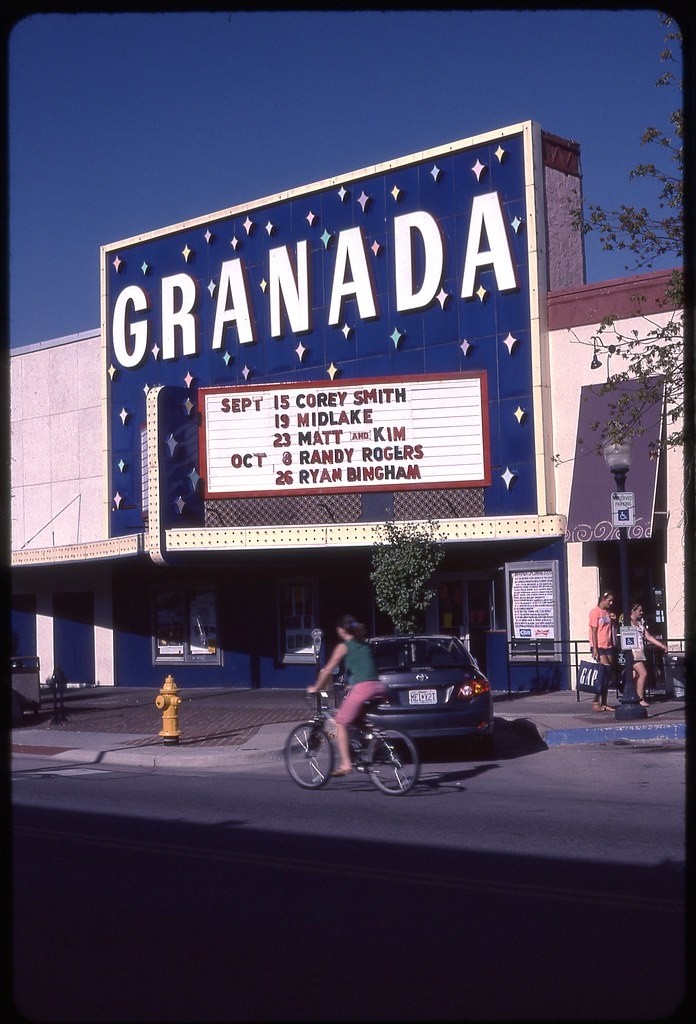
[576,656,610,694]
[632,648,646,661]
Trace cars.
[329,634,495,737]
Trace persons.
[306,615,389,777]
[589,591,617,712]
[622,603,668,707]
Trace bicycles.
[285,684,420,796]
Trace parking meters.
[310,628,324,714]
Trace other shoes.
[592,705,605,712]
[640,700,649,706]
[602,706,615,712]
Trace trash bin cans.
[9,655,42,716]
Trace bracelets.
[592,648,597,651]
[659,643,664,648]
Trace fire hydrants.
[155,675,183,747]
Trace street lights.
[601,420,649,722]
